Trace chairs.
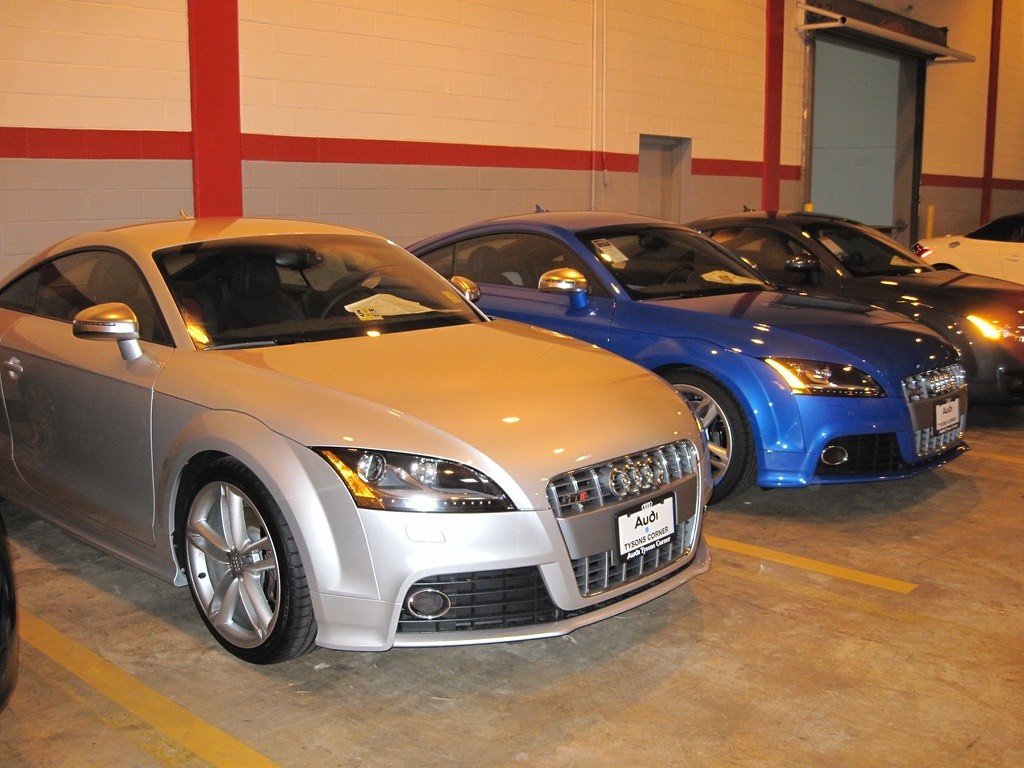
[70,256,154,342]
[761,237,792,270]
[222,254,308,329]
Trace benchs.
[445,245,558,288]
[36,253,224,326]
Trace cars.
[911,213,1024,287]
[0,215,709,665]
[677,213,1024,413]
[401,214,970,509]
